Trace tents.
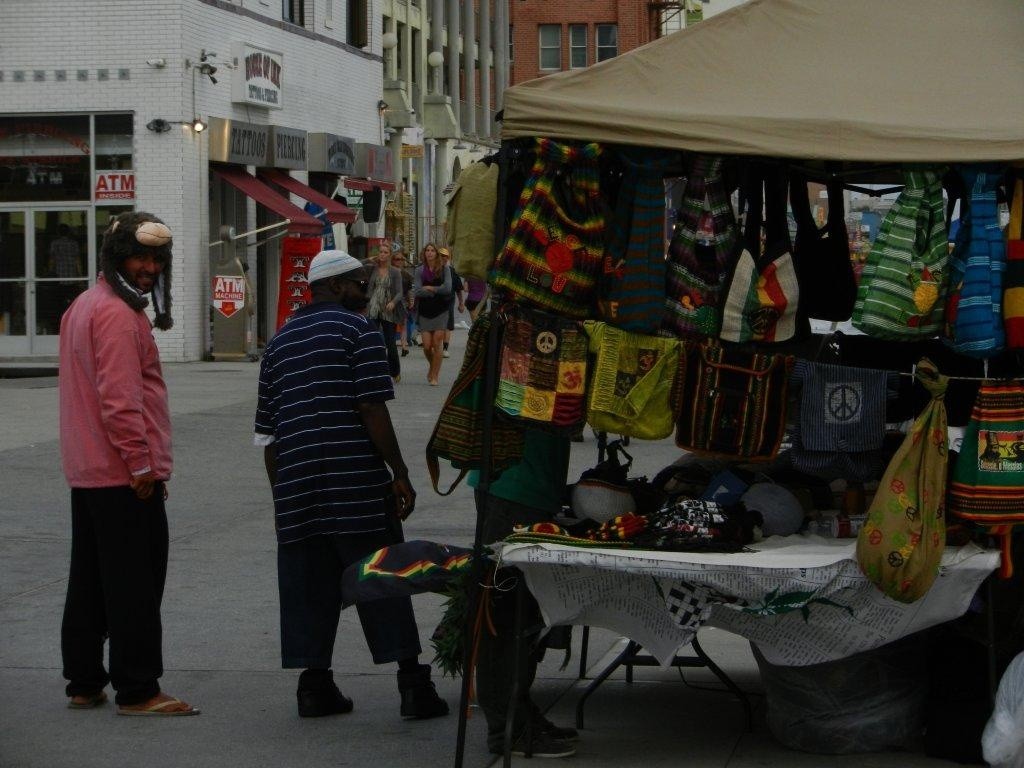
[449,0,1022,767]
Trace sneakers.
[491,716,578,760]
[401,349,409,357]
[443,349,449,358]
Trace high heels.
[427,372,437,385]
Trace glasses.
[338,275,370,296]
[440,254,447,257]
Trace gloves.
[392,376,401,383]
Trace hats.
[305,250,364,283]
[438,248,450,257]
[104,209,173,332]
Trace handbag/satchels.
[428,131,1022,598]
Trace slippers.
[65,692,107,710]
[116,690,199,716]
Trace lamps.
[379,99,388,110]
[382,31,398,61]
[452,131,495,158]
[198,48,219,85]
[427,50,444,79]
[419,129,438,147]
[192,120,207,133]
[382,114,397,134]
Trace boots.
[294,668,352,720]
[392,661,446,719]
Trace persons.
[469,434,585,760]
[255,249,450,723]
[461,275,489,321]
[361,243,404,385]
[438,249,466,357]
[59,211,206,716]
[391,253,413,356]
[413,242,453,385]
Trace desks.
[453,527,1024,768]
